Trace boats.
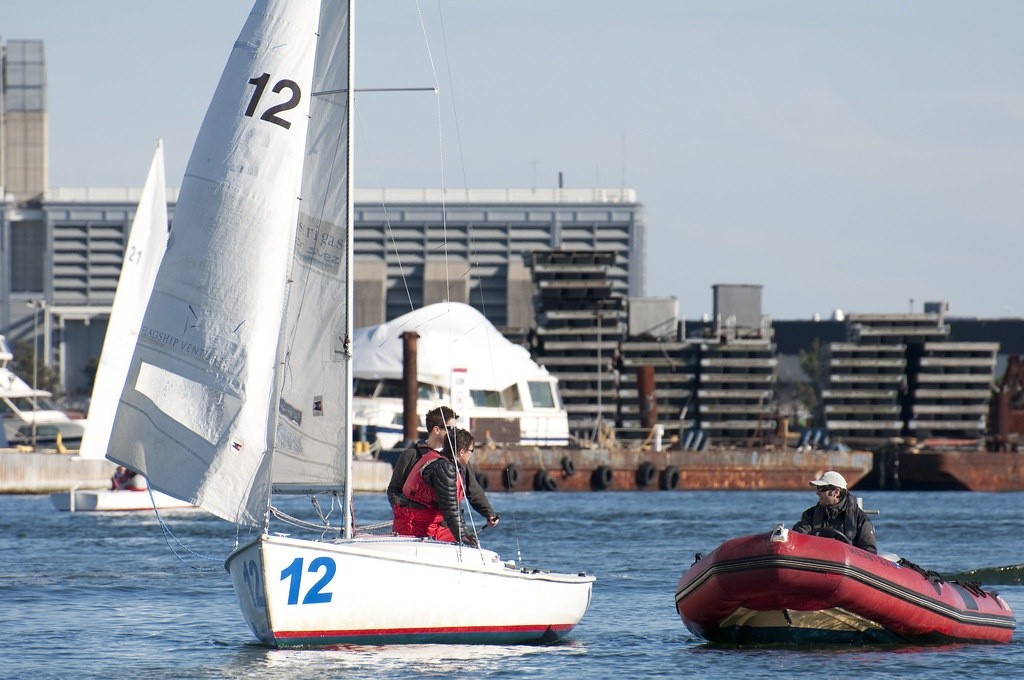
[350,397,470,493]
[0,333,87,450]
[675,525,1018,646]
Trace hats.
[809,471,847,491]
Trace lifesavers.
[537,469,559,492]
[476,473,492,491]
[597,465,613,486]
[562,457,576,474]
[662,465,680,490]
[637,461,656,488]
[505,465,521,487]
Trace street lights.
[27,298,46,452]
[592,310,603,417]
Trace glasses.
[467,448,474,453]
[440,426,457,432]
[816,487,831,492]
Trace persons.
[388,406,499,527]
[792,471,877,554]
[111,465,147,491]
[393,429,479,547]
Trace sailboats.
[51,139,207,514]
[104,0,596,649]
